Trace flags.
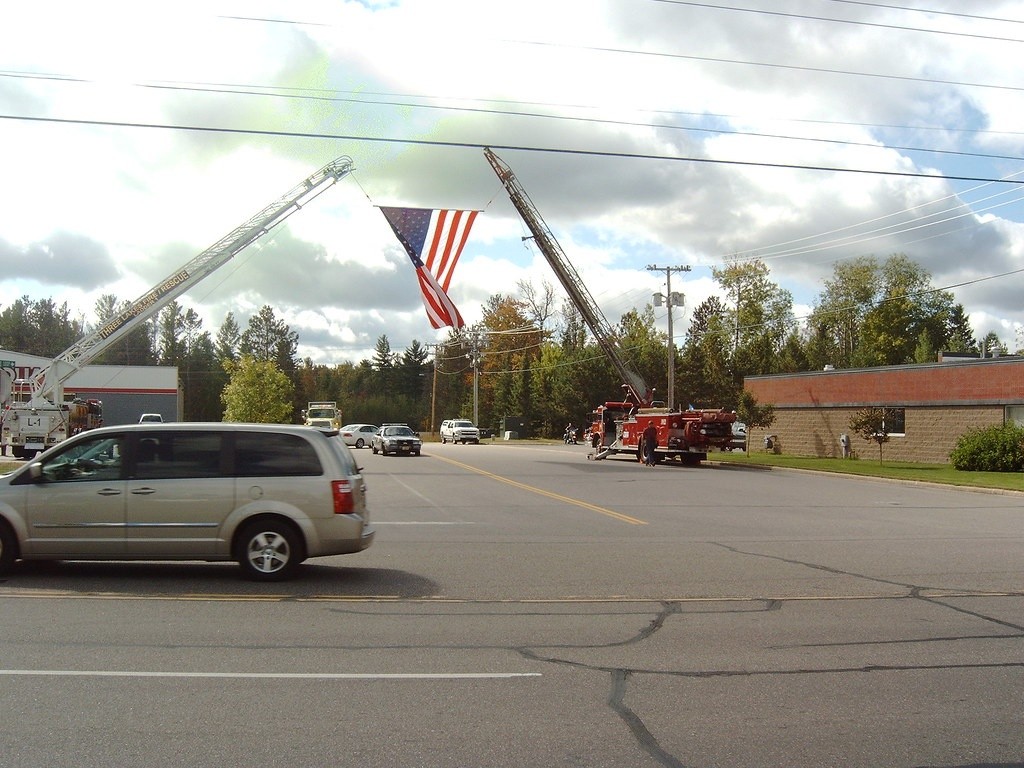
[379,205,481,330]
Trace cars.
[339,423,379,450]
[0,422,378,582]
[370,423,423,456]
[138,413,165,424]
[731,431,747,452]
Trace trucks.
[300,401,342,436]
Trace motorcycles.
[562,428,578,444]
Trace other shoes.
[647,463,655,467]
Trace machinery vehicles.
[0,155,353,461]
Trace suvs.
[440,418,480,446]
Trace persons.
[642,421,658,466]
[564,423,576,444]
[70,440,127,480]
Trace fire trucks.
[481,146,739,468]
